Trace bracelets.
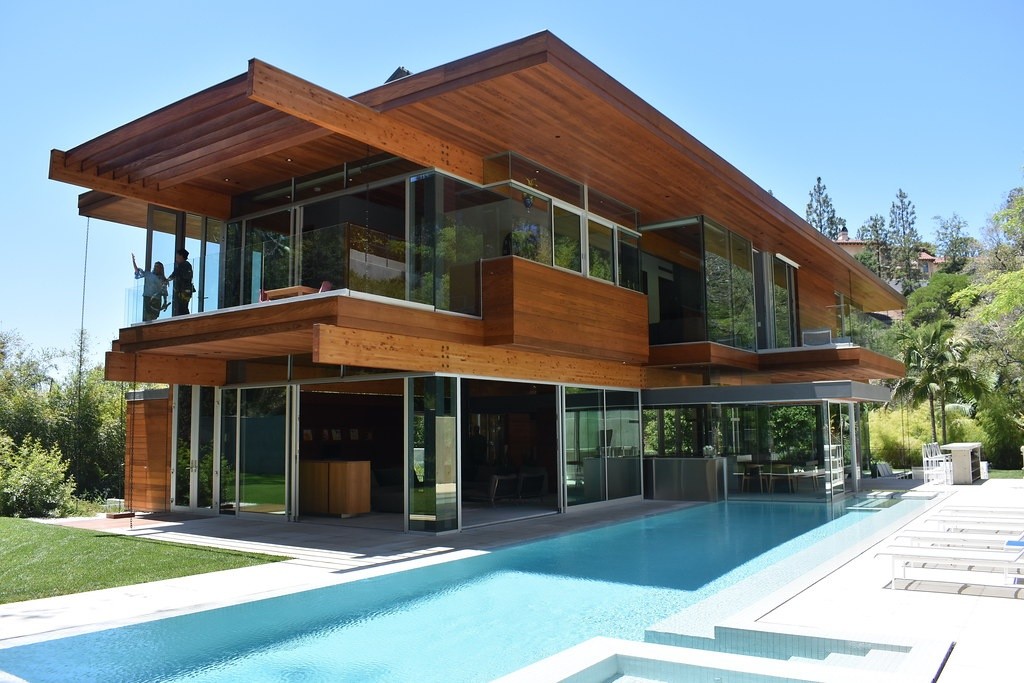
[168,277,171,281]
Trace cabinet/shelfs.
[939,442,981,485]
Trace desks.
[299,458,371,514]
[265,284,319,300]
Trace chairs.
[923,441,952,487]
[876,462,914,480]
[319,280,331,291]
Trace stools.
[770,464,794,493]
[742,463,769,495]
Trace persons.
[131,252,167,322]
[161,248,196,318]
